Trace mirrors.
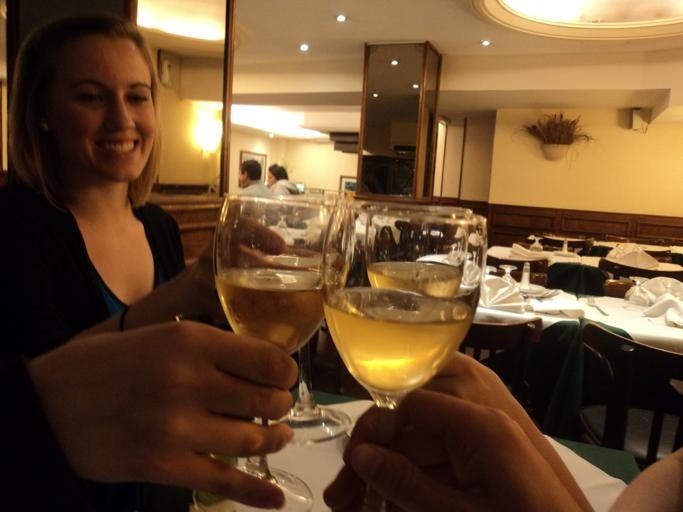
[352,39,443,204]
[124,1,235,210]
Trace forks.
[586,295,608,317]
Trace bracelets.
[118,307,128,333]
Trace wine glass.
[485,263,518,288]
[189,192,490,512]
[528,236,544,251]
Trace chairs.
[601,278,635,298]
[484,230,682,287]
[584,323,683,468]
[461,317,544,426]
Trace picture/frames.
[338,173,356,194]
[239,148,266,190]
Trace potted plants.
[512,113,594,165]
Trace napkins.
[631,277,681,307]
[478,277,526,314]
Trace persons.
[266,164,298,195]
[0,10,288,512]
[234,159,271,197]
[321,347,683,512]
[1,321,299,510]
